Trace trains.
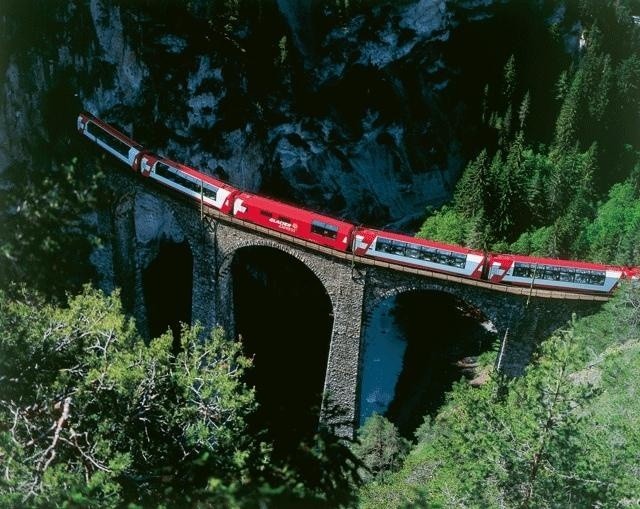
[74,112,639,302]
